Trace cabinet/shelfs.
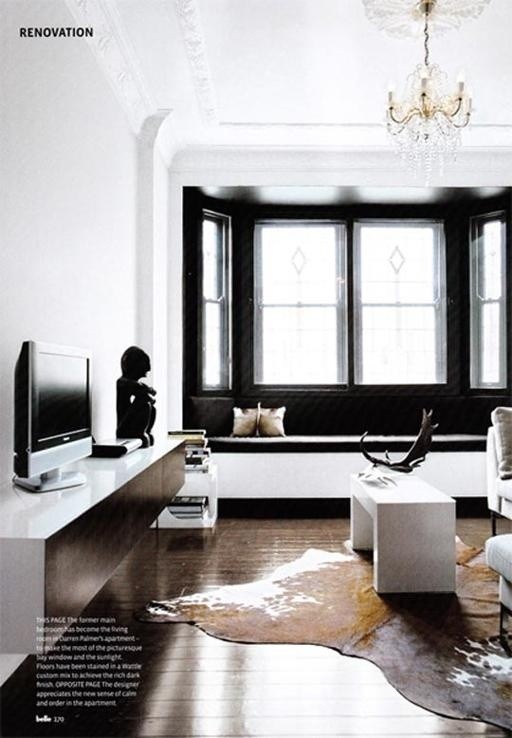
[150,461,218,529]
[0,438,185,655]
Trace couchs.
[486,408,512,535]
[183,397,502,518]
[485,534,512,659]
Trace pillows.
[259,406,286,436]
[232,407,258,436]
[492,407,511,478]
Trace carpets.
[134,535,512,729]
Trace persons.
[114,345,157,448]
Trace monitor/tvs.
[14,340,93,490]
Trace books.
[166,429,210,474]
[168,496,206,520]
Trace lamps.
[362,1,491,189]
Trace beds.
[346,472,456,594]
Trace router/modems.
[92,436,142,458]
